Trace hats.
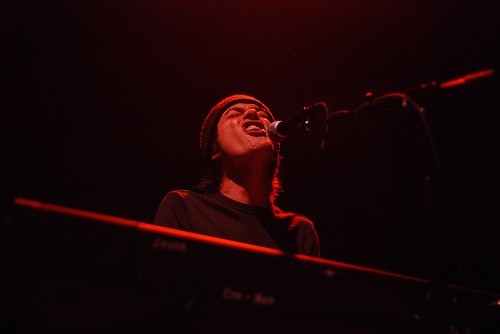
[200,93,275,152]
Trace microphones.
[268,103,323,141]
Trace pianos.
[0,198,500,334]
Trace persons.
[150,94,319,302]
